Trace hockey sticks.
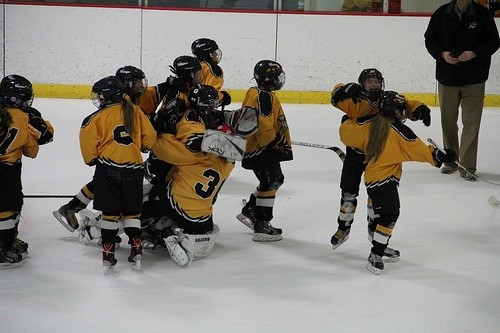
[290,140,346,162]
[488,195,500,207]
[426,137,500,186]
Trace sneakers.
[13,237,29,259]
[77,209,101,245]
[102,242,118,275]
[128,237,142,271]
[252,220,283,242]
[53,195,87,233]
[330,216,354,250]
[163,235,189,266]
[0,248,23,270]
[382,246,400,263]
[236,193,257,230]
[365,248,385,275]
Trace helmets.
[189,85,219,111]
[116,66,146,96]
[91,76,123,102]
[378,91,408,118]
[0,74,32,102]
[253,60,282,91]
[191,38,218,63]
[358,69,384,89]
[168,55,202,80]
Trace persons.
[331,68,431,259]
[424,0,500,181]
[79,75,157,268]
[0,74,54,264]
[241,60,294,236]
[341,0,382,12]
[58,38,259,266]
[338,90,459,271]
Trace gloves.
[413,104,431,128]
[339,82,368,104]
[432,148,458,167]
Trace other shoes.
[440,165,477,181]
